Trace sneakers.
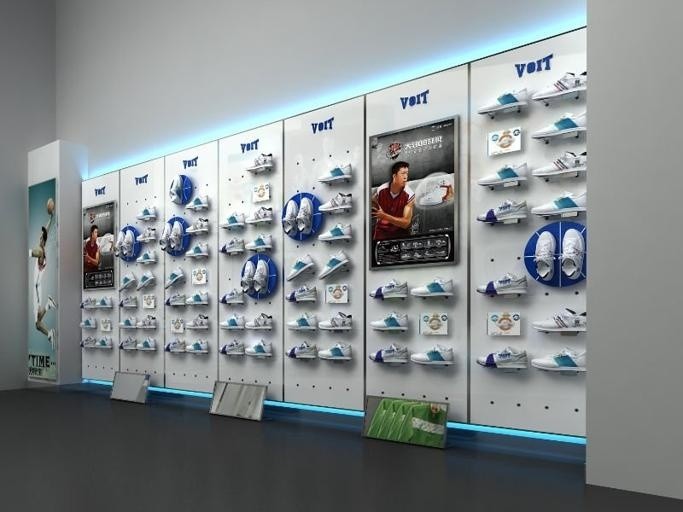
[160,218,209,257]
[532,152,586,177]
[476,272,527,294]
[369,279,408,299]
[282,197,313,236]
[246,153,272,173]
[285,250,353,360]
[370,312,409,330]
[80,297,112,349]
[46,296,57,311]
[532,72,587,100]
[477,162,527,186]
[317,164,352,241]
[476,346,528,368]
[411,344,455,365]
[410,277,453,297]
[219,207,273,253]
[531,347,587,371]
[164,268,184,289]
[478,88,528,114]
[531,308,587,331]
[535,231,556,281]
[114,208,157,350]
[218,288,245,303]
[369,342,409,363]
[169,175,183,205]
[241,260,269,294]
[530,112,587,138]
[476,199,527,222]
[185,195,208,210]
[165,293,211,353]
[531,190,587,215]
[561,229,585,279]
[47,328,55,352]
[219,313,274,356]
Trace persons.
[28,212,58,351]
[83,225,101,272]
[370,160,417,241]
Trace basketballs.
[47,198,55,213]
